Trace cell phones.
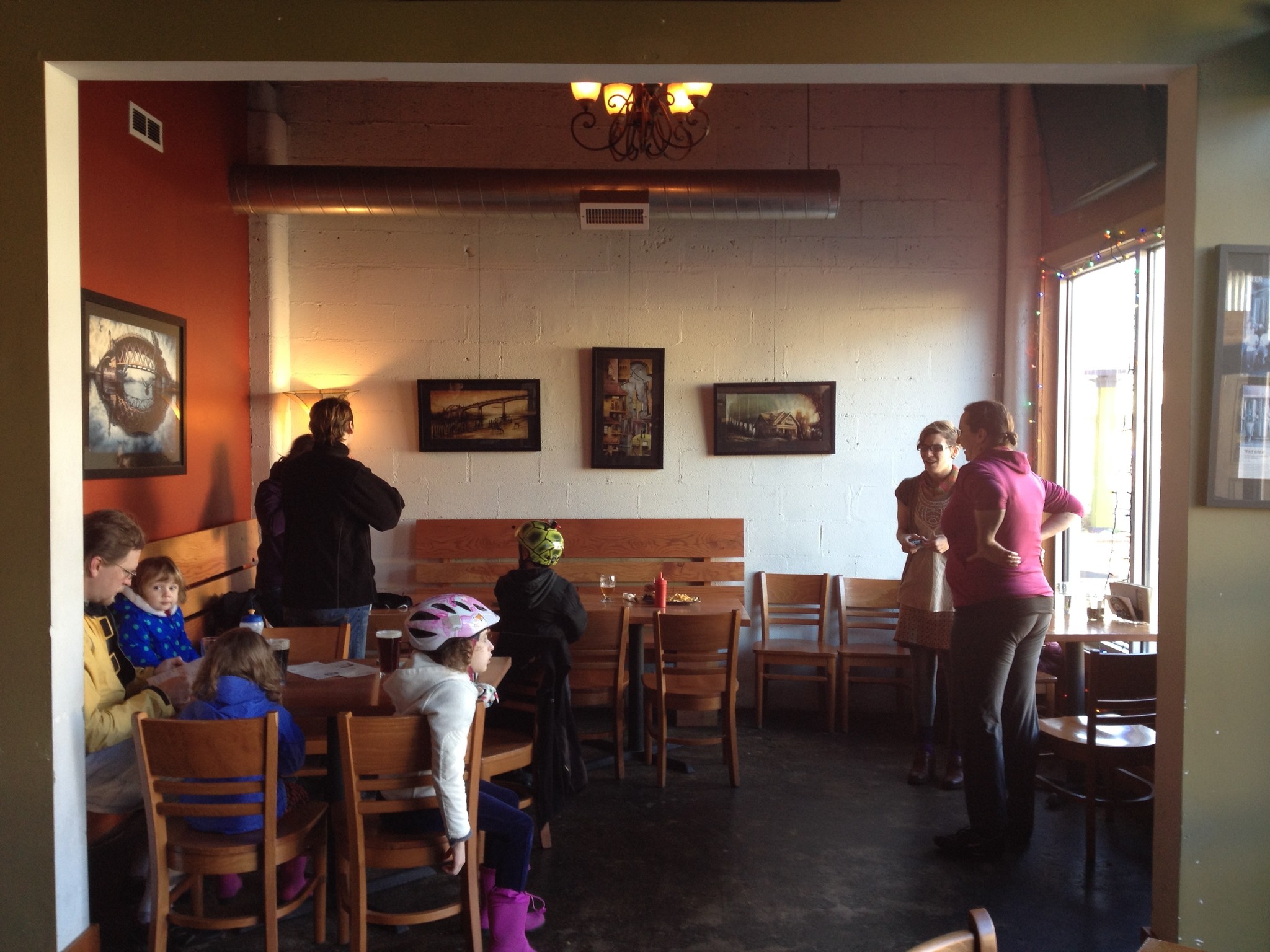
[911,540,924,548]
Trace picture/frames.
[714,382,836,456]
[590,347,665,469]
[80,288,186,480]
[1204,246,1270,509]
[417,379,541,453]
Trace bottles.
[239,609,264,635]
[654,572,667,608]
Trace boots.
[277,856,314,899]
[488,886,546,952]
[213,874,243,905]
[478,862,546,933]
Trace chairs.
[1034,650,1157,864]
[483,629,552,849]
[641,609,740,788]
[752,571,839,730]
[242,614,405,664]
[131,700,328,952]
[563,606,630,779]
[334,700,486,952]
[836,576,918,734]
[1036,670,1057,718]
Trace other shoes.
[934,826,1000,853]
[1002,834,1031,852]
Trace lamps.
[283,390,359,414]
[569,83,712,161]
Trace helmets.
[513,520,565,567]
[404,593,500,652]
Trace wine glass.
[600,574,616,603]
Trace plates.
[641,596,698,604]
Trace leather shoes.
[907,751,934,785]
[943,756,964,789]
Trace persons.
[111,555,198,669]
[895,422,959,789]
[1242,321,1270,369]
[937,402,1084,860]
[493,524,588,789]
[180,629,312,902]
[381,594,546,952]
[83,511,188,924]
[255,397,404,657]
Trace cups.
[266,639,291,686]
[376,630,403,680]
[201,637,218,657]
[1086,595,1105,622]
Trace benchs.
[414,519,746,665]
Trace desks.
[1045,615,1157,717]
[401,595,752,772]
[174,657,512,948]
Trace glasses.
[956,427,979,437]
[101,558,137,579]
[917,442,952,451]
